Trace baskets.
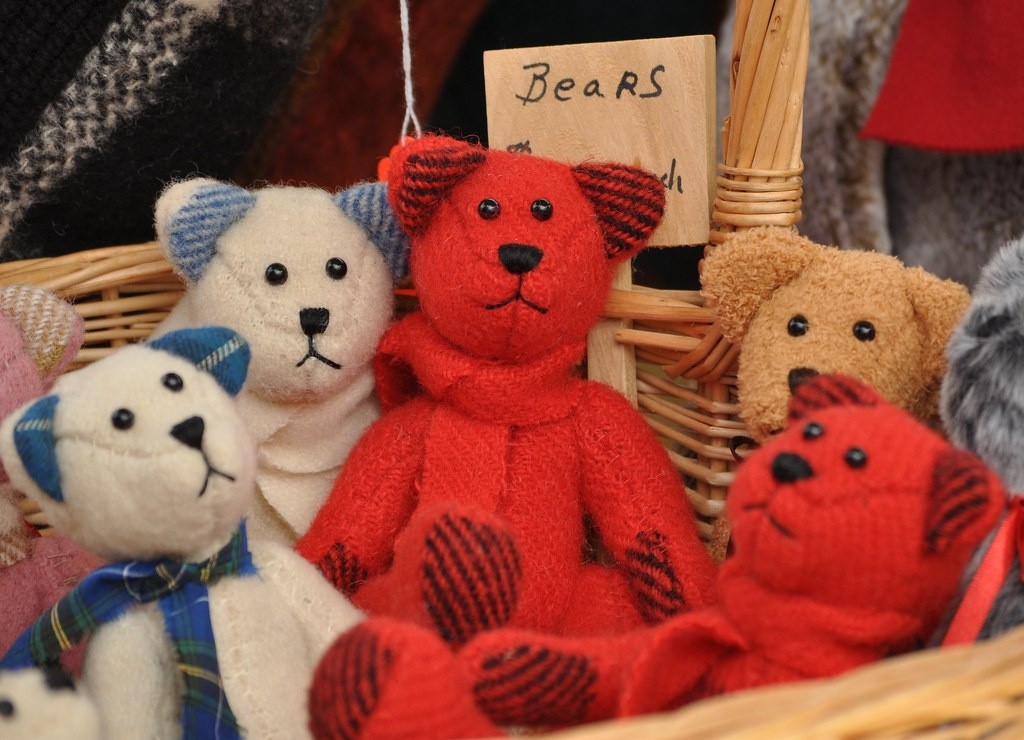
[0,0,1022,739]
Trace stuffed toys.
[703,226,974,567]
[156,179,427,548]
[0,286,111,675]
[0,325,370,740]
[296,137,720,650]
[308,372,1024,740]
[0,666,101,740]
[939,235,1024,639]
